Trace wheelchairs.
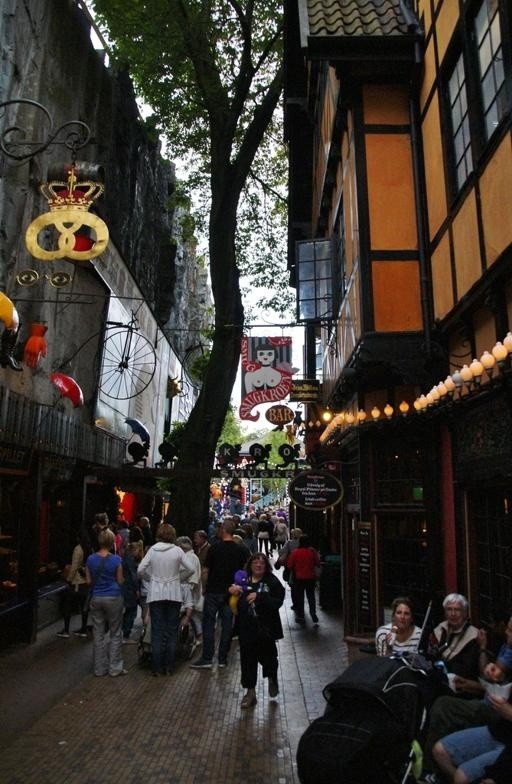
[135,609,197,660]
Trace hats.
[291,528,302,537]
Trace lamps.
[284,330,512,444]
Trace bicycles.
[52,308,157,399]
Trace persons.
[274,529,321,624]
[375,595,424,662]
[415,614,512,783]
[432,672,512,784]
[56,503,289,679]
[423,591,481,682]
[480,687,512,783]
[228,551,285,709]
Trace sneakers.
[219,660,226,667]
[241,695,256,708]
[122,640,138,644]
[73,629,88,637]
[269,681,278,696]
[55,628,69,637]
[189,658,212,668]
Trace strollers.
[293,648,442,783]
